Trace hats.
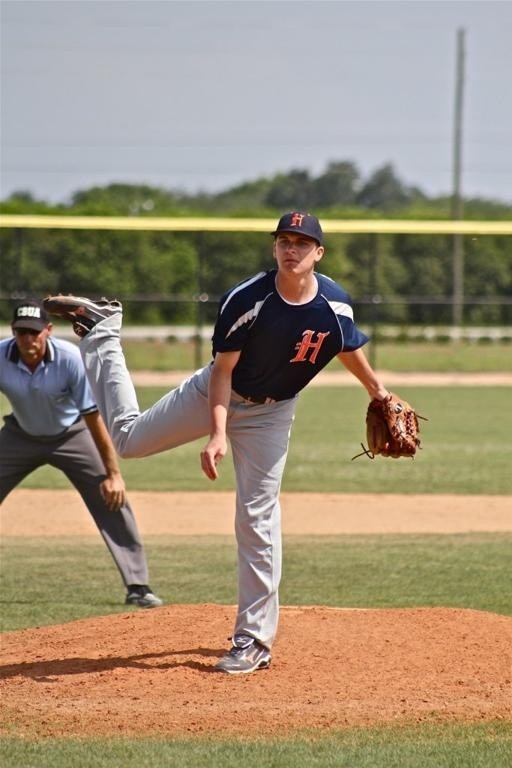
[269,212,322,247]
[10,302,49,332]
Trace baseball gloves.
[365,392,419,457]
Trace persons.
[0,301,166,608]
[41,210,430,674]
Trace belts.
[210,362,266,404]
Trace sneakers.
[215,633,272,674]
[126,584,162,608]
[43,293,123,337]
